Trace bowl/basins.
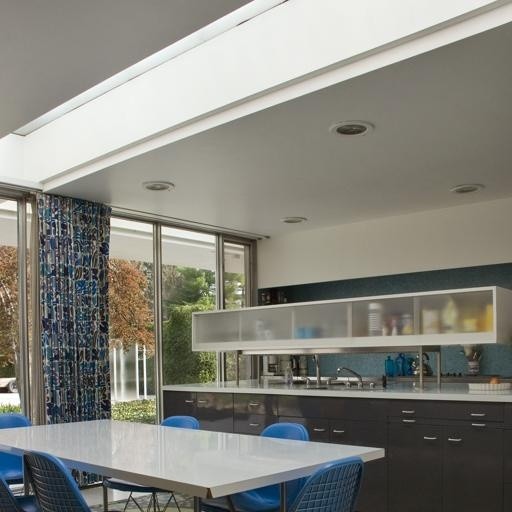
[294,327,321,338]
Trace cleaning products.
[285,362,294,390]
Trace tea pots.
[409,352,432,375]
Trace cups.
[468,361,479,377]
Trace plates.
[468,391,511,394]
[467,383,510,391]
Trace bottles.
[298,355,308,376]
[236,319,274,340]
[367,301,414,337]
[284,363,293,391]
[384,352,415,377]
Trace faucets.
[337,367,363,390]
[312,353,320,388]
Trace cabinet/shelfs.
[163,391,512,511]
[191,284,512,351]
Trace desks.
[1,419,387,512]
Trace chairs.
[230,422,310,512]
[21,451,92,512]
[102,415,200,512]
[0,473,39,512]
[0,413,34,485]
[287,456,365,512]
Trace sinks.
[303,386,385,392]
